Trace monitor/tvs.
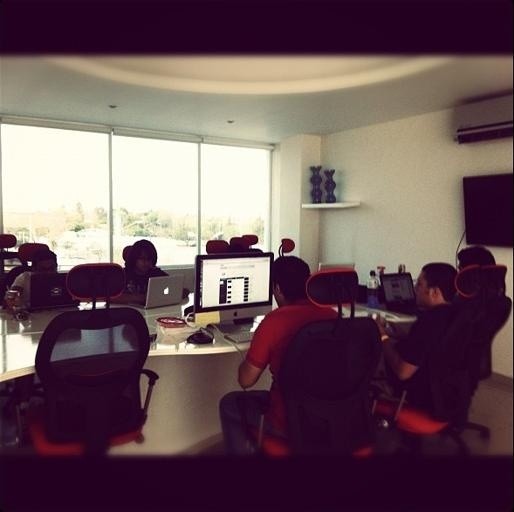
[462,172,514,249]
[194,252,273,333]
[316,262,355,273]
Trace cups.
[5,291,20,316]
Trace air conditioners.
[451,93,513,144]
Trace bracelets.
[379,332,390,343]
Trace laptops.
[129,275,185,310]
[25,273,81,310]
[379,272,421,315]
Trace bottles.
[366,270,380,307]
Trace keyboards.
[224,332,256,343]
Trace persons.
[220,253,341,459]
[0,249,58,310]
[456,246,497,274]
[353,261,460,461]
[109,239,188,305]
